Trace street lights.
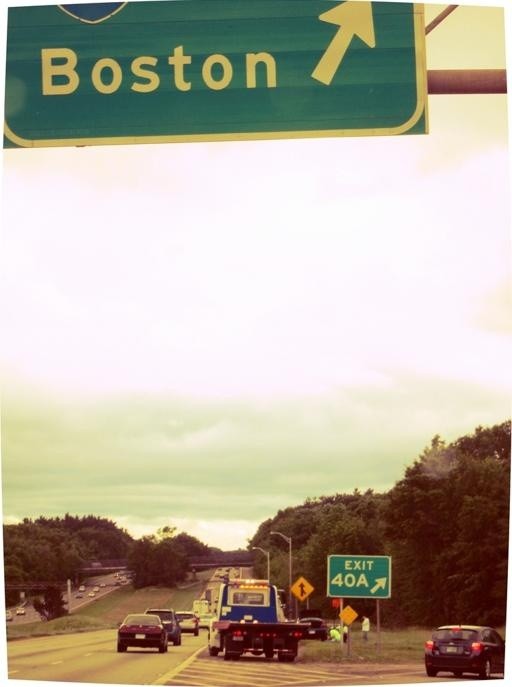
[270,531,292,621]
[252,546,270,580]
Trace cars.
[213,567,240,579]
[6,608,13,622]
[75,583,106,599]
[111,570,132,586]
[15,607,27,616]
[424,624,505,679]
[291,617,330,641]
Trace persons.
[344,625,348,642]
[361,615,371,639]
[329,626,341,642]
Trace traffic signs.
[2,0,430,147]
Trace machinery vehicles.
[117,600,213,653]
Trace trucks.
[208,577,310,662]
